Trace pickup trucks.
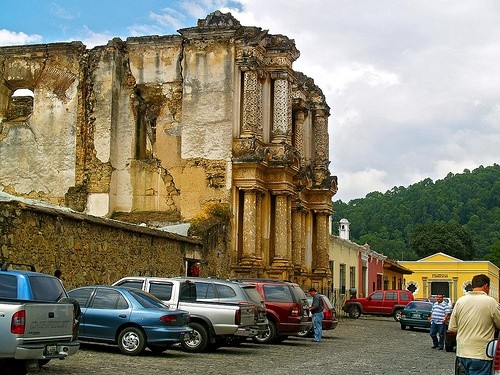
[105,278,242,353]
[0,300,80,362]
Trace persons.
[53,270,61,278]
[447,274,500,375]
[429,293,451,350]
[308,288,324,342]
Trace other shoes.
[310,338,320,342]
[431,345,444,350]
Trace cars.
[65,285,194,356]
[304,293,339,337]
[400,301,433,330]
[443,312,457,352]
[415,297,453,309]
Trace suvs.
[232,278,312,344]
[164,276,269,347]
[342,289,415,322]
[0,262,81,365]
